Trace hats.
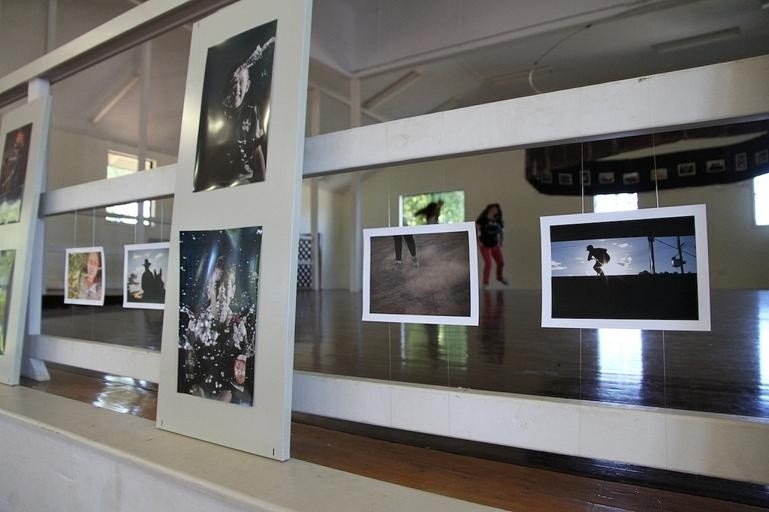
[142,259,151,266]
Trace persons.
[394,234,421,270]
[187,255,251,405]
[4,130,26,193]
[141,258,154,292]
[586,245,610,282]
[80,251,101,301]
[473,202,509,289]
[211,62,265,184]
